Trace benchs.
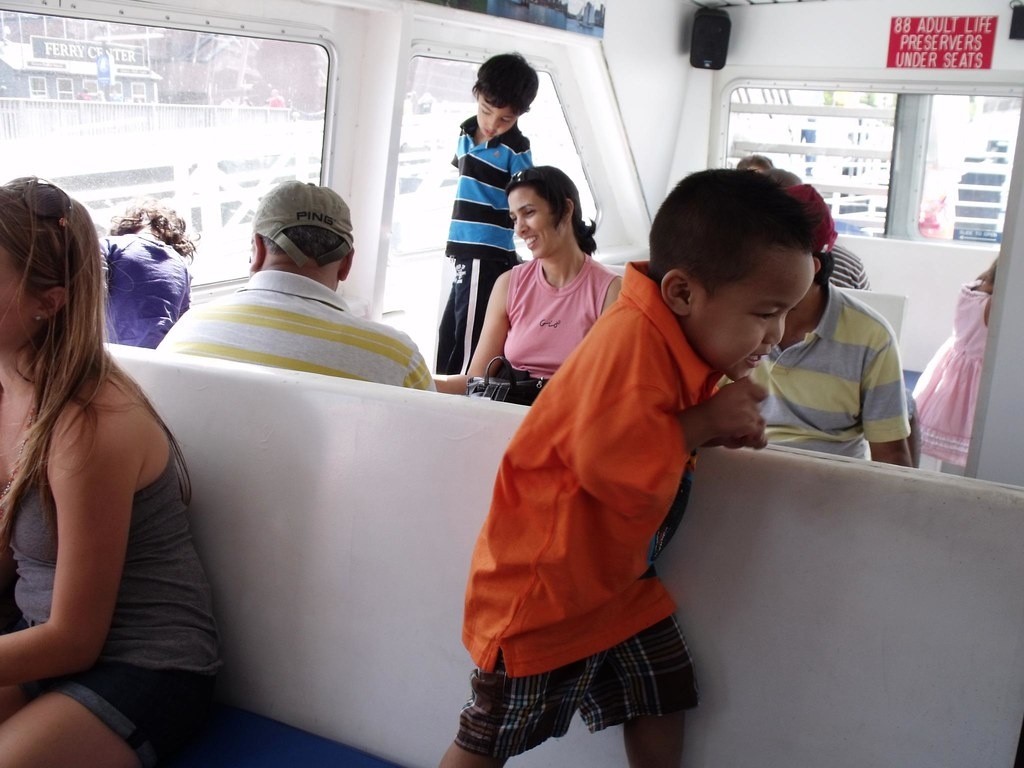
[96,233,1024,768]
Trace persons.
[432,50,540,376]
[431,166,623,395]
[736,155,872,292]
[912,256,1001,466]
[98,199,201,350]
[0,177,223,768]
[156,179,436,393]
[438,170,815,767]
[715,185,912,468]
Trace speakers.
[689,7,730,69]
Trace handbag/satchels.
[467,356,542,407]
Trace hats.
[782,183,839,254]
[251,180,355,268]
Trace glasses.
[510,168,546,186]
[9,178,74,278]
